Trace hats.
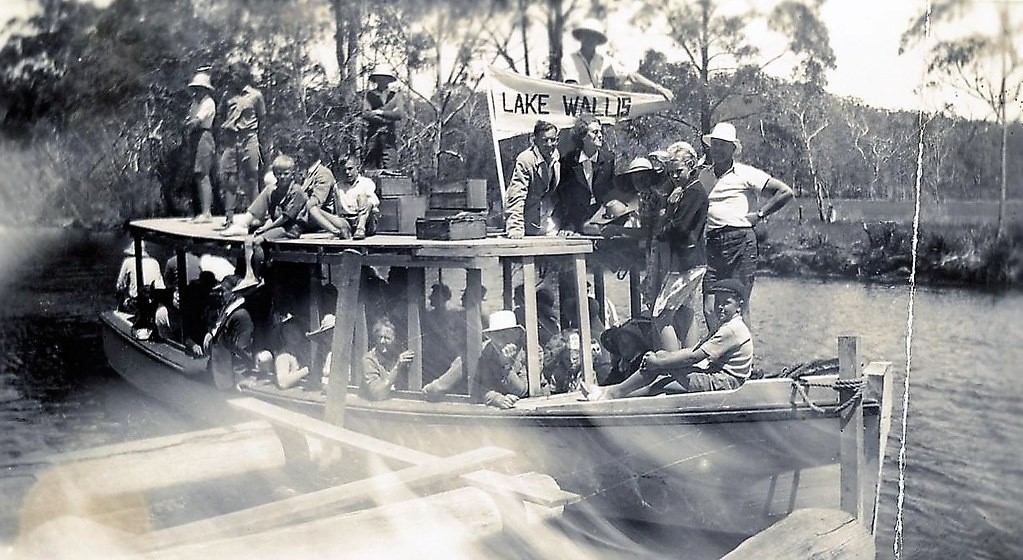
[369,62,398,81]
[617,157,664,191]
[188,72,216,90]
[482,310,526,336]
[707,278,746,302]
[123,238,149,257]
[305,314,336,343]
[702,122,743,154]
[591,199,635,225]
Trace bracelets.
[655,83,660,92]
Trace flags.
[484,66,672,140]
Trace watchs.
[757,209,765,219]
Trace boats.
[100,214,880,530]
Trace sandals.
[353,228,366,240]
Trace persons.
[558,19,675,159]
[117,62,794,408]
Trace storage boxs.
[373,170,490,241]
